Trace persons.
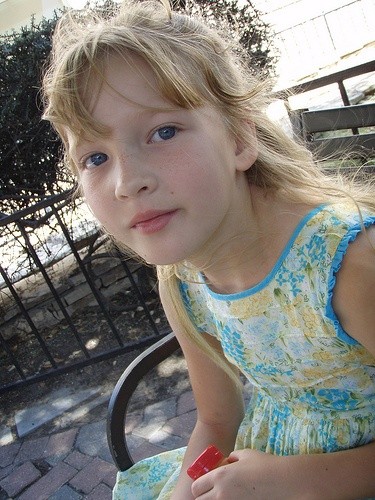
[40,0,375,500]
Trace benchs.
[106,102,374,471]
